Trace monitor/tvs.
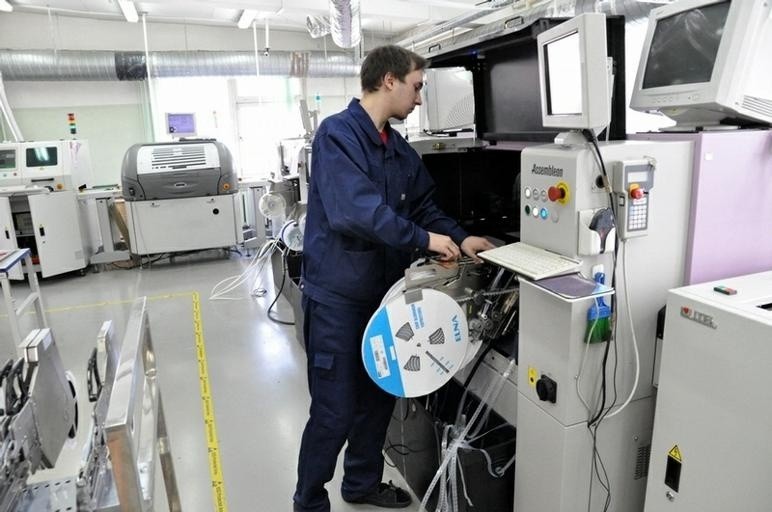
[20,142,63,182]
[166,112,198,141]
[404,65,474,137]
[536,11,610,148]
[629,0,766,130]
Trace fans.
[259,191,287,239]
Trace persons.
[291,44,497,510]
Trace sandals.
[354,480,411,508]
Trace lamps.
[237,9,258,29]
[118,0,138,23]
[0,0,13,14]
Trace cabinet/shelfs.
[0,193,86,280]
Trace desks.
[0,248,47,350]
